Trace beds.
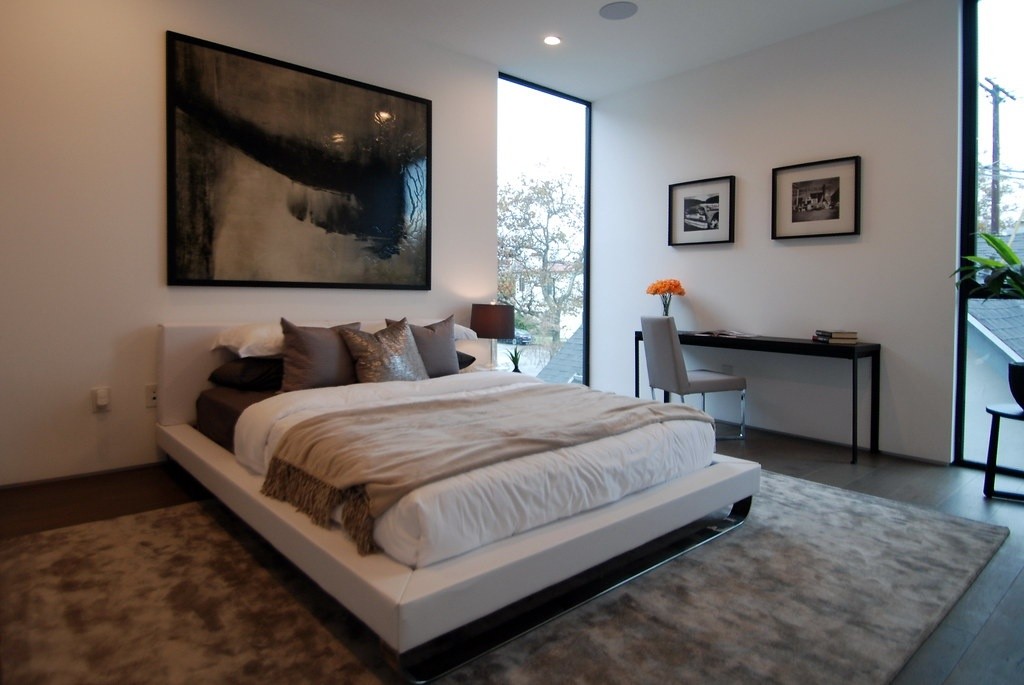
[156,320,762,684]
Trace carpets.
[0,466,1010,685]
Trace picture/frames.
[772,155,861,239]
[166,30,432,290]
[668,175,734,246]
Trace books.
[812,330,858,344]
[694,330,761,338]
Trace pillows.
[341,317,430,382]
[206,354,284,391]
[281,316,361,390]
[412,318,478,341]
[209,324,286,358]
[385,314,460,377]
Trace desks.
[634,330,881,465]
[982,405,1024,501]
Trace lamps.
[470,303,515,368]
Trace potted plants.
[949,232,1024,411]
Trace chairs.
[641,316,747,440]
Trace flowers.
[646,277,686,316]
